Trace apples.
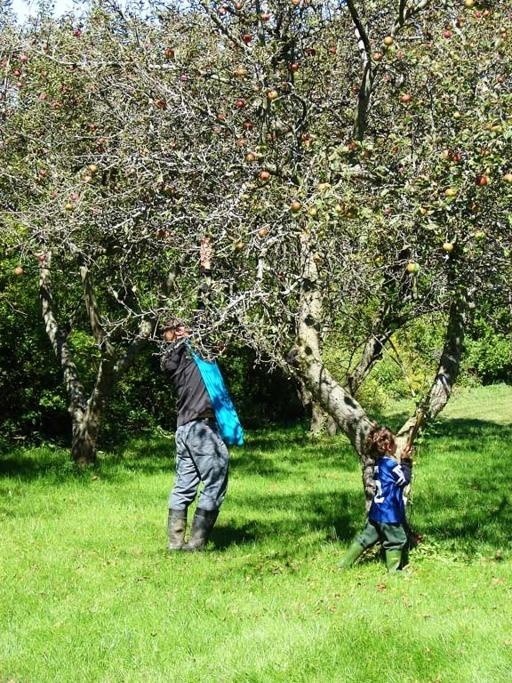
[14,1,512,276]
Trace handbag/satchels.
[194,356,244,447]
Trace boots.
[332,541,364,569]
[385,548,404,575]
[168,507,220,551]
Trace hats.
[161,318,184,330]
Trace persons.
[154,235,230,555]
[336,427,413,575]
